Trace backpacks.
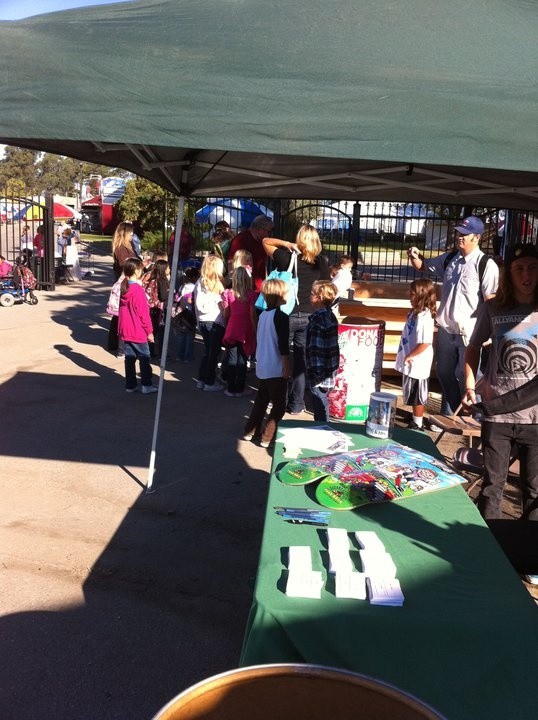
[105,280,124,317]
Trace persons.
[0,213,99,297]
[393,278,439,431]
[462,243,538,586]
[407,216,500,433]
[106,214,355,361]
[115,257,160,394]
[304,278,340,424]
[489,209,538,261]
[192,254,226,392]
[222,265,258,396]
[243,276,290,447]
[262,222,329,415]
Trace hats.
[504,243,538,268]
[452,216,484,235]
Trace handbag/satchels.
[254,250,300,316]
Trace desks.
[239,416,537,720]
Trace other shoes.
[244,433,261,441]
[203,381,226,392]
[235,389,255,397]
[126,385,141,393]
[177,358,192,363]
[141,385,158,393]
[406,421,425,431]
[525,574,538,584]
[117,352,126,358]
[259,440,275,448]
[430,424,443,432]
[196,379,204,389]
[290,406,307,415]
[267,402,274,408]
[402,413,413,423]
[223,388,235,397]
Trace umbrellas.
[192,197,274,231]
[11,201,83,222]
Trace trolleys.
[0,263,38,307]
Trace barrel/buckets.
[365,391,398,440]
[148,663,445,719]
[329,318,386,422]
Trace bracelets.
[464,388,477,392]
